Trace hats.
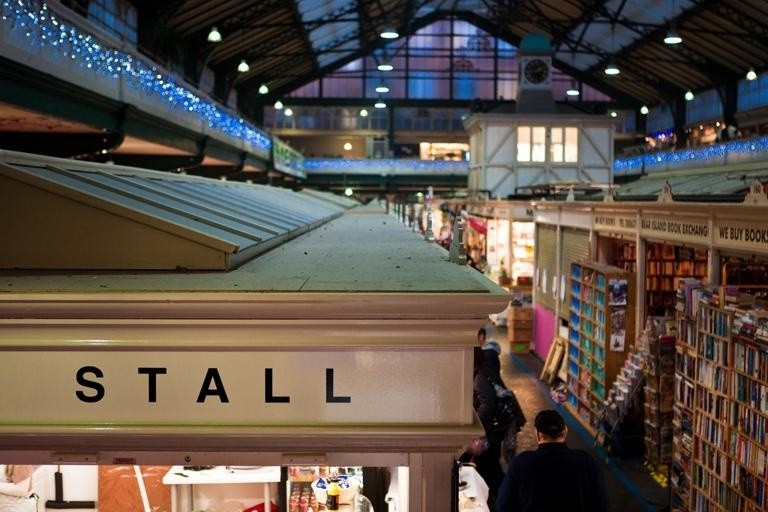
[535,410,564,435]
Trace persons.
[458,454,490,512]
[473,327,516,467]
[495,410,609,511]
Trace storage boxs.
[507,306,535,355]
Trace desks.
[162,465,283,512]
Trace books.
[567,244,767,511]
